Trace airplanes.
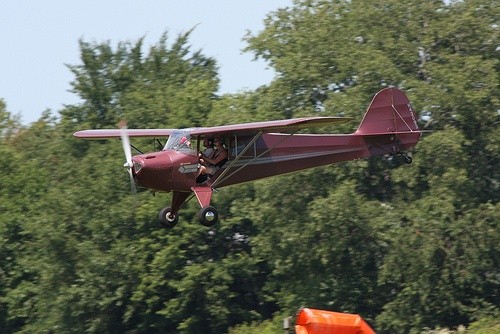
[72,86,432,229]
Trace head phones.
[206,138,212,144]
[215,136,225,144]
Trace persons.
[193,134,227,183]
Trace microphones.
[203,145,206,147]
[214,144,216,146]
